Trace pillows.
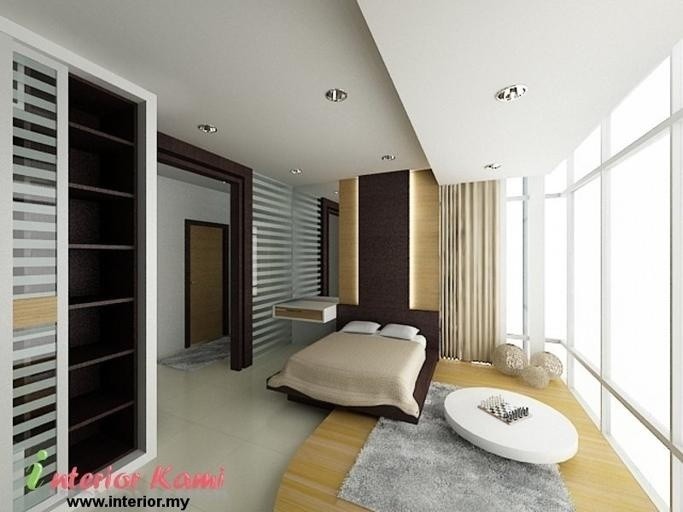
[341,319,420,341]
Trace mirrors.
[290,181,339,298]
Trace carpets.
[333,380,577,512]
[158,337,230,373]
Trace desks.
[271,299,337,324]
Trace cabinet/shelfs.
[0,30,147,512]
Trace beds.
[264,302,439,424]
[443,385,577,464]
